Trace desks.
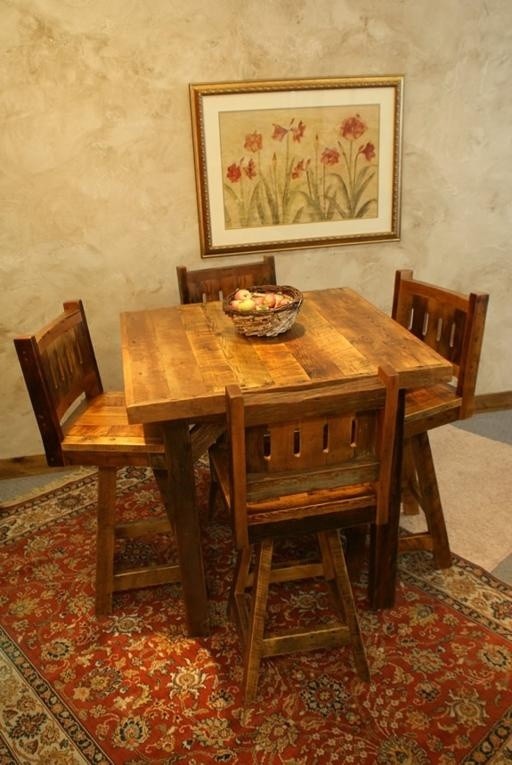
[119,286,453,638]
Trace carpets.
[2,457,511,765]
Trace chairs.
[392,270,489,569]
[209,365,401,706]
[177,254,277,304]
[14,299,227,616]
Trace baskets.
[222,284,301,338]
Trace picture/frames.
[185,73,405,259]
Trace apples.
[229,289,291,312]
